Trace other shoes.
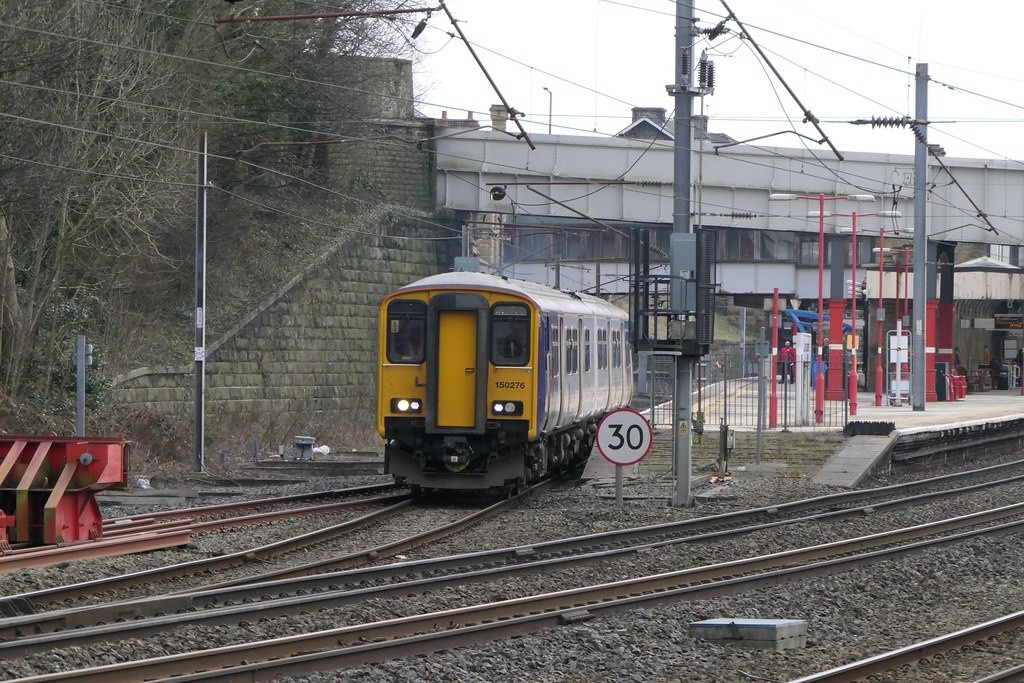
[790,380,794,384]
[778,381,784,383]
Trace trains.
[378,270,634,502]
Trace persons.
[778,341,796,384]
[822,338,829,361]
[954,347,968,382]
[1016,349,1024,386]
[989,356,1003,389]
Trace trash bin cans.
[998,372,1008,390]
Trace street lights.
[871,247,911,404]
[840,226,916,405]
[768,194,876,420]
[806,210,903,415]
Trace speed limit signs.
[596,409,653,466]
